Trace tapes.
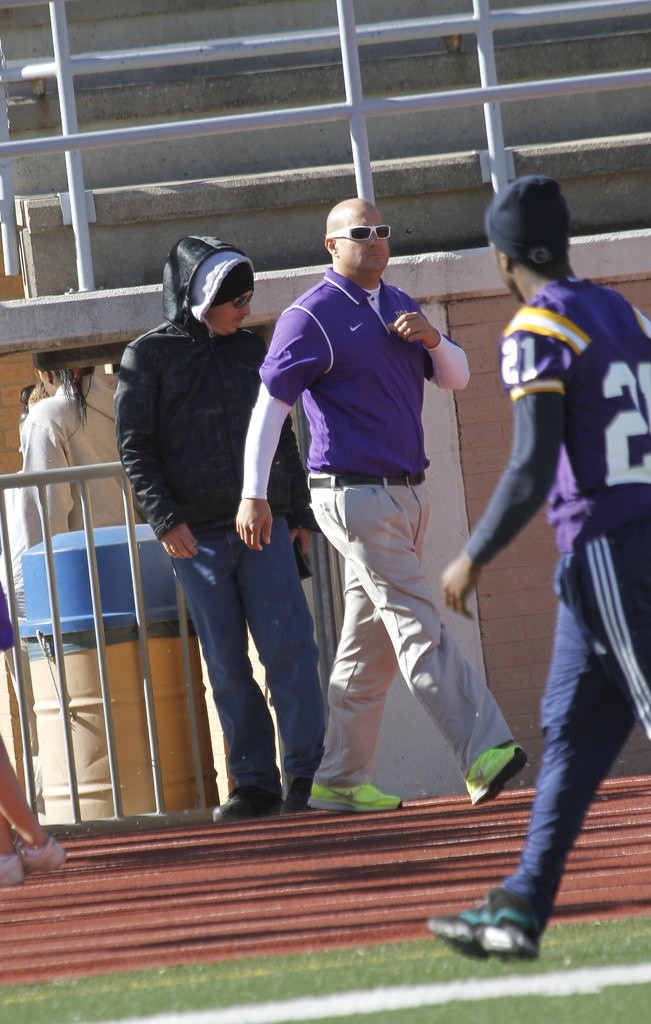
[294,537,313,579]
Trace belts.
[309,470,426,489]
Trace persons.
[20,364,143,551]
[113,237,326,823]
[237,198,528,815]
[0,582,65,887]
[426,175,651,962]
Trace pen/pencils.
[195,545,214,557]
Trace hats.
[485,176,572,263]
[211,262,254,307]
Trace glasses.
[233,294,253,307]
[326,225,391,241]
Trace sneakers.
[427,887,540,962]
[283,777,314,810]
[212,787,283,821]
[0,855,25,887]
[19,837,69,876]
[465,745,528,805]
[308,782,403,811]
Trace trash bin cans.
[18,525,221,823]
